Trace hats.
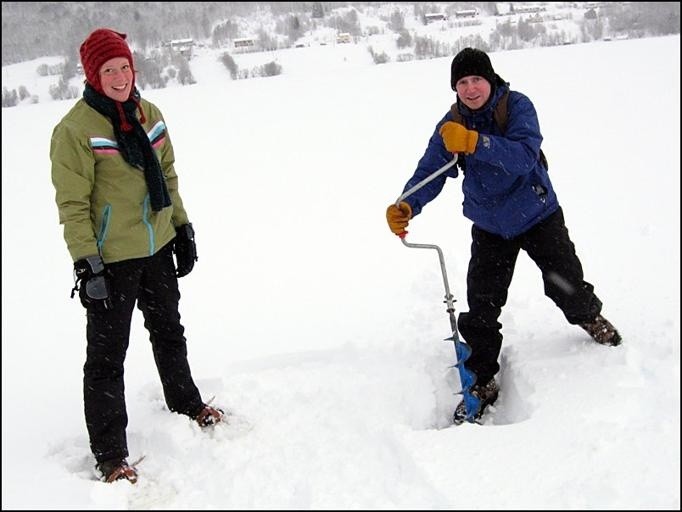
[450,46,496,92]
[79,29,136,104]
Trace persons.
[50,28,226,486]
[385,47,621,424]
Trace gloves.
[173,222,198,277]
[385,200,413,235]
[71,254,118,314]
[438,121,479,155]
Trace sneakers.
[581,313,622,347]
[454,376,499,421]
[194,402,222,429]
[94,455,138,485]
